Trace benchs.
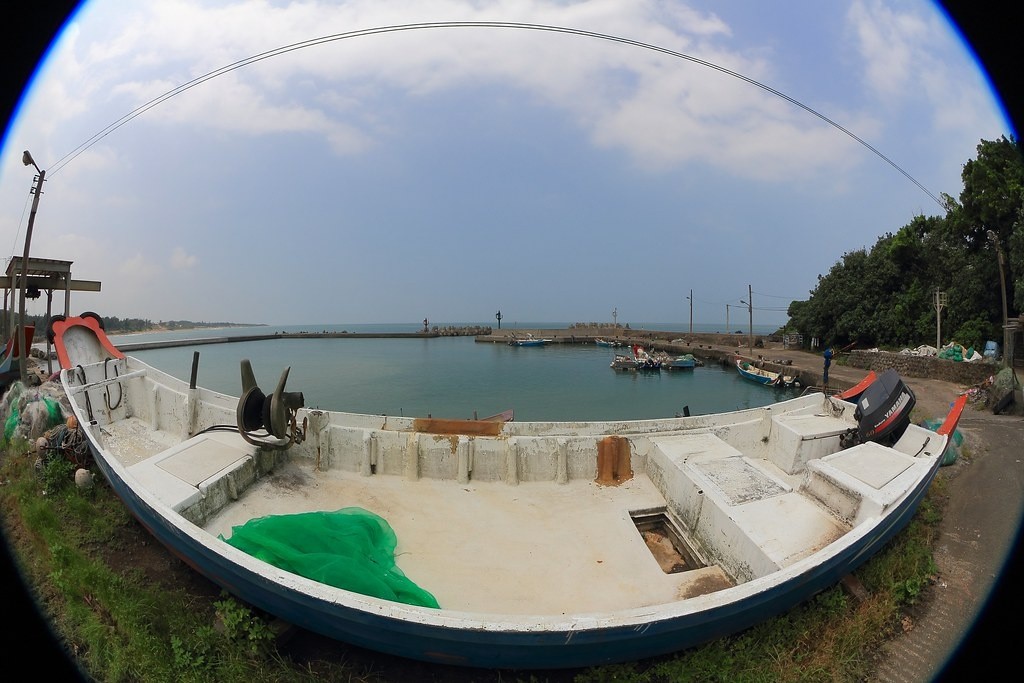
[644,432,851,586]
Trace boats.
[610,343,705,372]
[736,359,800,388]
[47,311,970,671]
[594,337,623,348]
[508,332,552,346]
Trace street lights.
[740,284,753,356]
[19,150,46,395]
[686,289,693,334]
[986,229,1008,372]
